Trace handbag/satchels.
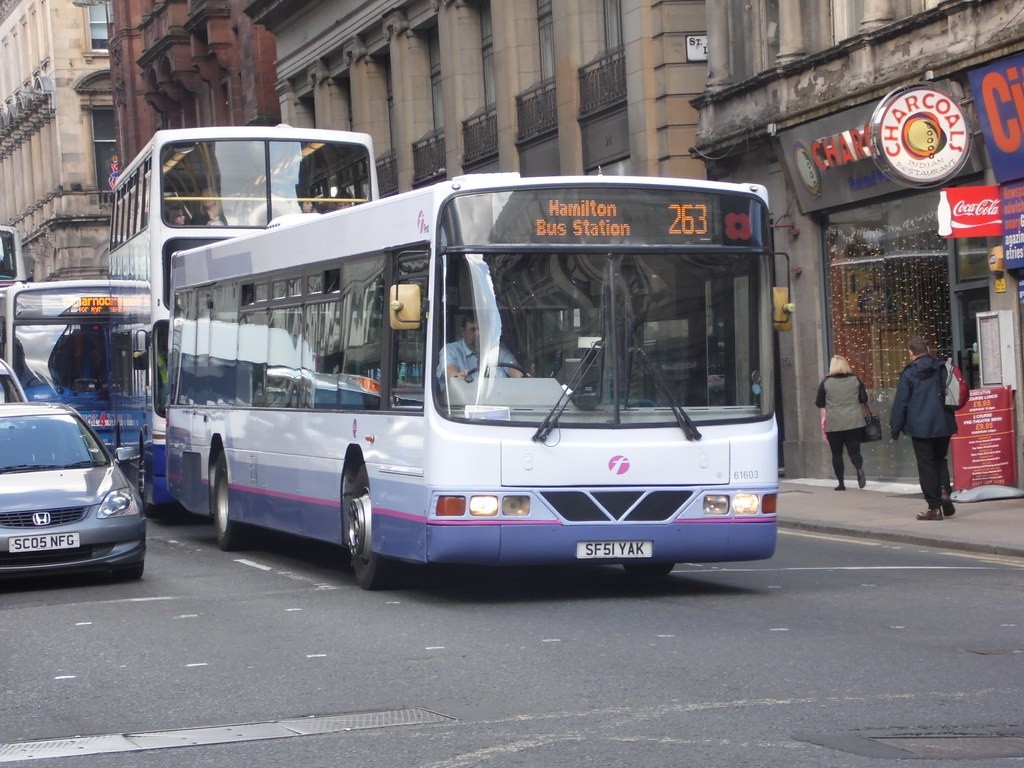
[860,398,882,442]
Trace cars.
[0,357,30,407]
[0,401,148,588]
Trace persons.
[298,193,314,213]
[813,353,870,491]
[190,187,233,227]
[335,192,352,211]
[887,335,959,521]
[164,207,189,226]
[434,309,524,394]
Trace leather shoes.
[941,485,955,516]
[917,509,943,519]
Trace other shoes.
[835,486,845,490]
[857,469,866,488]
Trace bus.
[109,126,383,518]
[0,225,28,335]
[0,277,152,493]
[164,171,782,598]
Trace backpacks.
[937,356,969,410]
